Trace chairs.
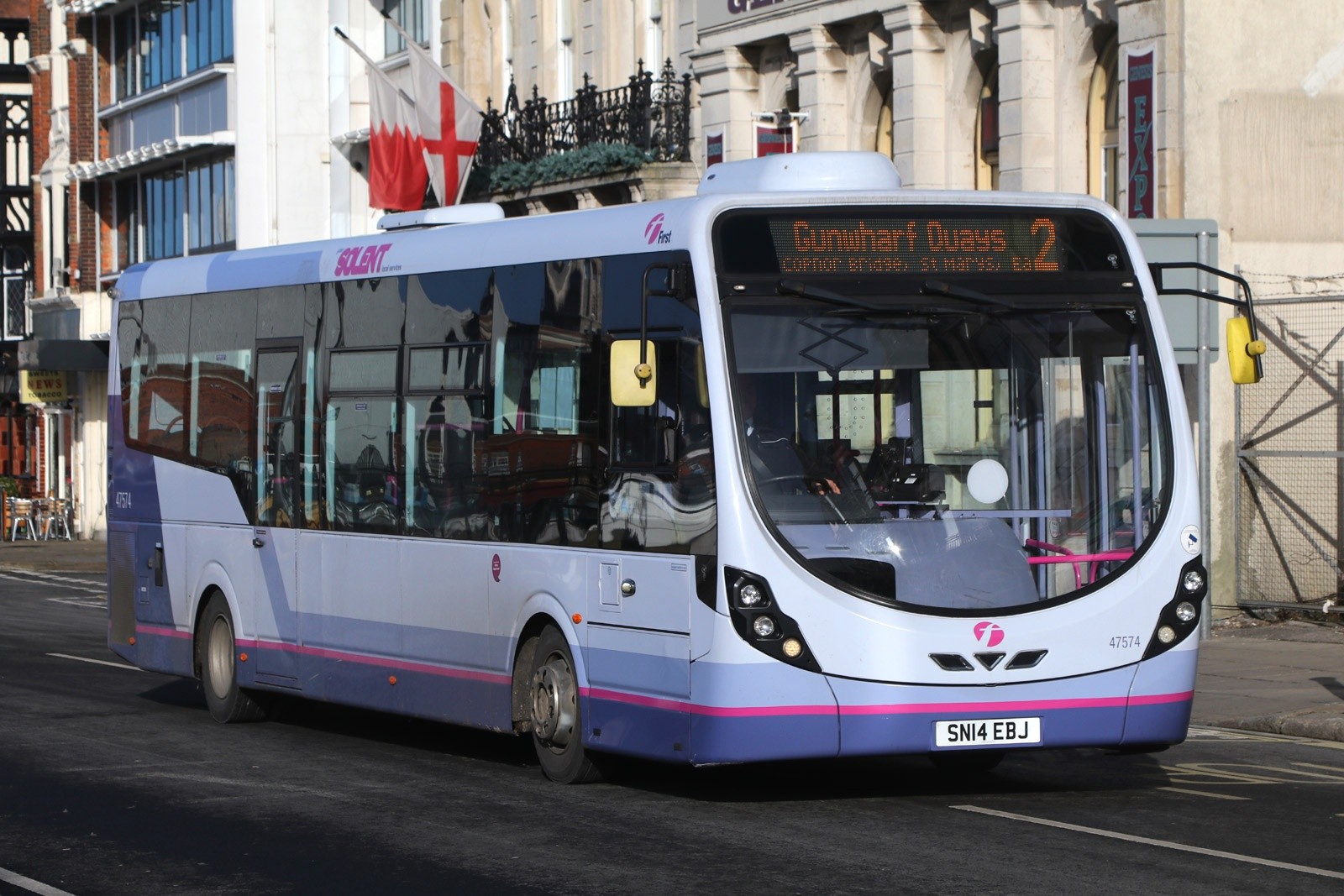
[7,497,75,543]
[160,412,518,547]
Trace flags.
[369,64,427,210]
[404,36,485,206]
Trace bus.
[99,150,1268,787]
